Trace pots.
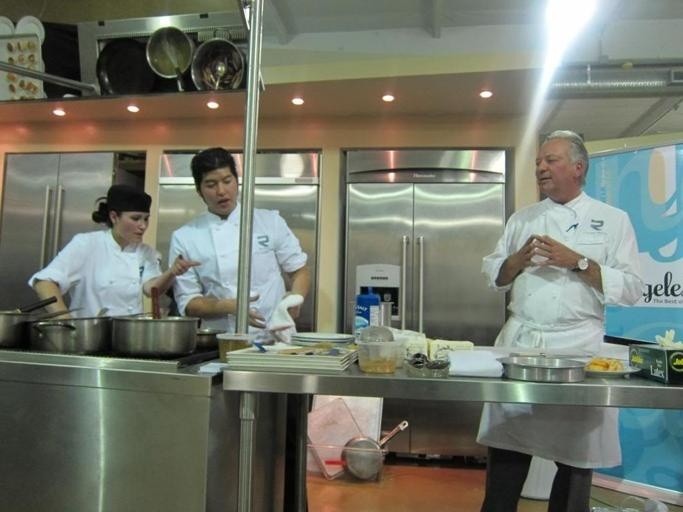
[146,25,192,79]
[0,309,48,351]
[112,313,201,359]
[95,36,159,97]
[341,420,409,482]
[191,28,245,93]
[26,316,110,357]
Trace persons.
[27,185,201,320]
[169,147,311,512]
[480,130,643,512]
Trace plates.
[0,16,15,40]
[289,332,356,346]
[586,364,642,378]
[226,344,358,372]
[12,15,47,46]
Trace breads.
[587,357,623,372]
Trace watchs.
[572,256,589,272]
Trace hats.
[108,185,151,211]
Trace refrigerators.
[160,152,321,347]
[0,154,142,310]
[342,149,507,467]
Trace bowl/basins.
[196,329,227,349]
[216,333,254,362]
[354,340,401,373]
[496,354,588,383]
